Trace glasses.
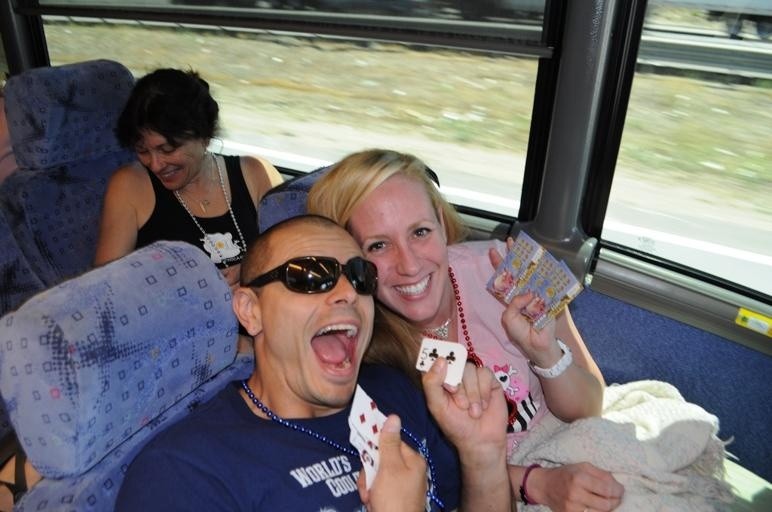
[246,256,378,295]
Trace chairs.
[0,60,136,314]
[257,167,333,235]
[0,241,256,511]
